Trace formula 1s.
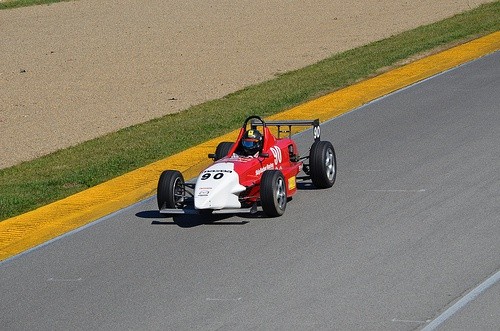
[157,116,338,217]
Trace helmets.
[242,130,262,153]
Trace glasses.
[241,139,261,149]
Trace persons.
[230,129,262,159]
